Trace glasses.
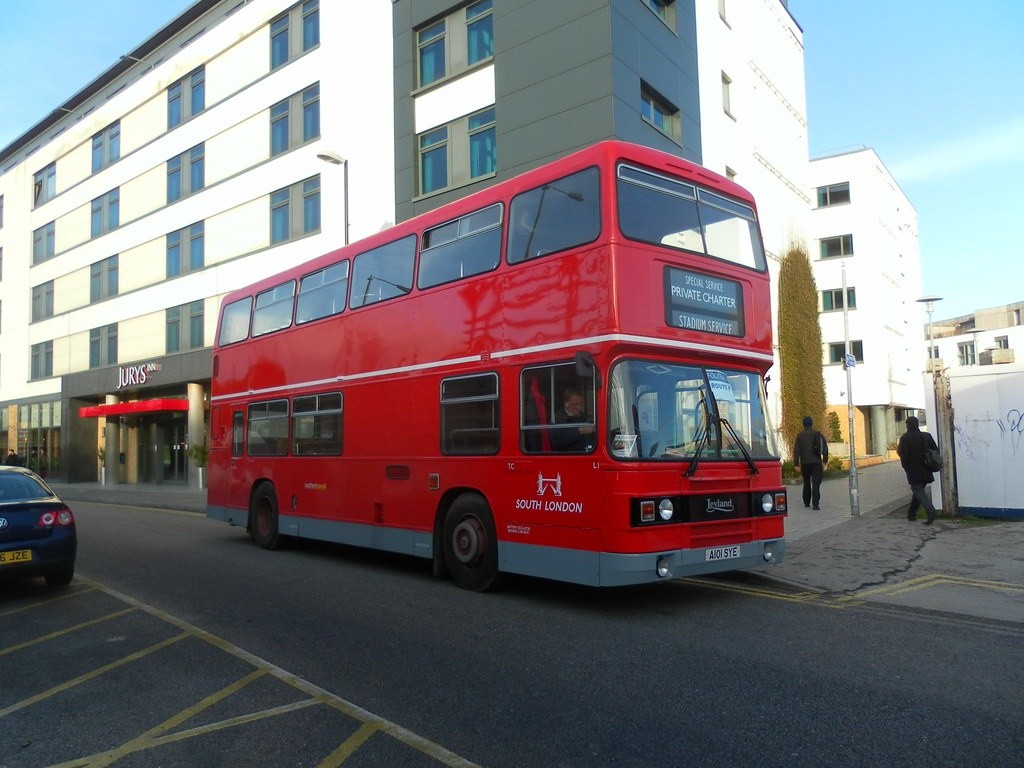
[567,402,583,408]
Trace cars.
[1,465,78,595]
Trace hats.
[905,416,919,424]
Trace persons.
[794,417,828,510]
[895,416,940,525]
[5,449,19,467]
[548,387,596,452]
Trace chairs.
[525,374,552,452]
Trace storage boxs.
[979,348,1014,365]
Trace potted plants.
[96,446,106,486]
[183,439,207,489]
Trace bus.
[207,138,789,594]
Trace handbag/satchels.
[814,431,823,454]
[923,432,943,472]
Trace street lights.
[917,294,943,380]
[965,327,985,368]
[314,148,350,248]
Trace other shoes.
[922,512,936,524]
[908,516,916,521]
[813,507,819,510]
